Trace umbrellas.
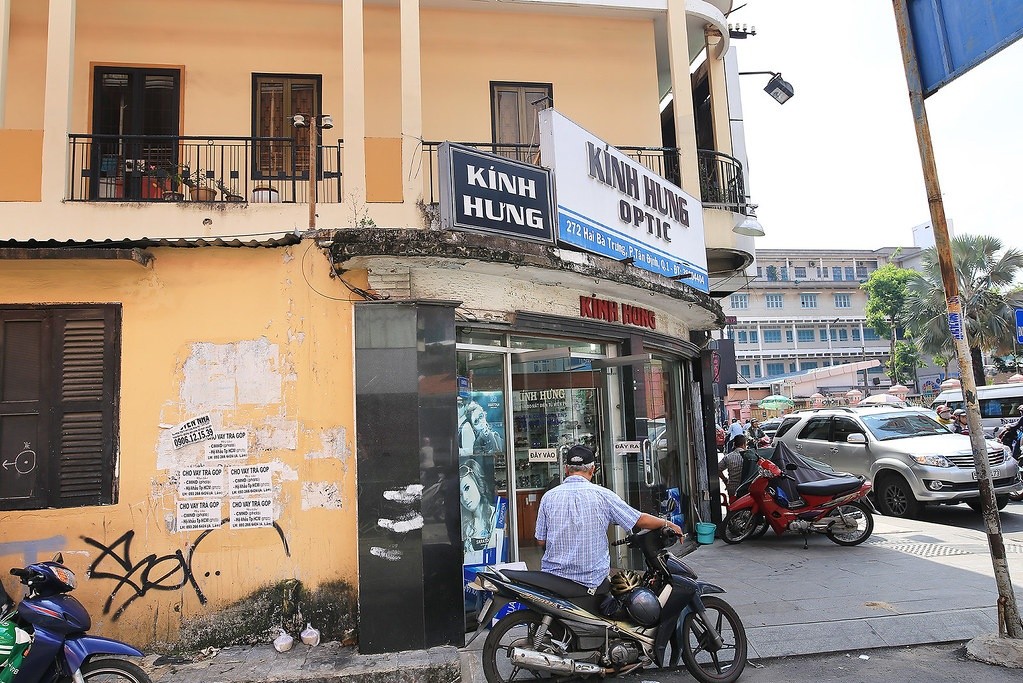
[757,395,794,411]
[858,394,906,407]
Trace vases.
[161,190,183,201]
[226,195,244,202]
[190,188,216,202]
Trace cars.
[716,424,726,452]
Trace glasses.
[471,413,483,427]
[458,466,474,478]
[494,397,599,488]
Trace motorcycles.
[0,552,152,683]
[719,451,881,546]
[463,525,747,683]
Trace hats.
[566,445,595,465]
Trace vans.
[928,382,1023,438]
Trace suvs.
[760,403,1023,519]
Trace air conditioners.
[858,261,866,266]
[809,262,816,267]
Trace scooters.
[962,427,1023,501]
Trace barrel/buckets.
[695,523,716,544]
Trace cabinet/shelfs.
[499,490,549,547]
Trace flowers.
[210,178,232,196]
[148,165,178,192]
[166,159,207,188]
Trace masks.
[942,412,952,420]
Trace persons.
[718,435,750,504]
[460,459,505,563]
[534,447,684,676]
[747,418,770,448]
[936,404,970,436]
[1004,405,1023,467]
[722,418,744,454]
[456,390,505,456]
[742,419,751,430]
[419,436,435,469]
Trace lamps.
[702,201,766,236]
[728,23,756,36]
[740,71,794,105]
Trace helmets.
[936,405,951,414]
[751,418,757,423]
[625,590,660,627]
[953,409,967,416]
[732,418,737,422]
[0,620,18,665]
[609,569,643,595]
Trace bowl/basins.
[274,638,293,652]
[301,632,319,645]
